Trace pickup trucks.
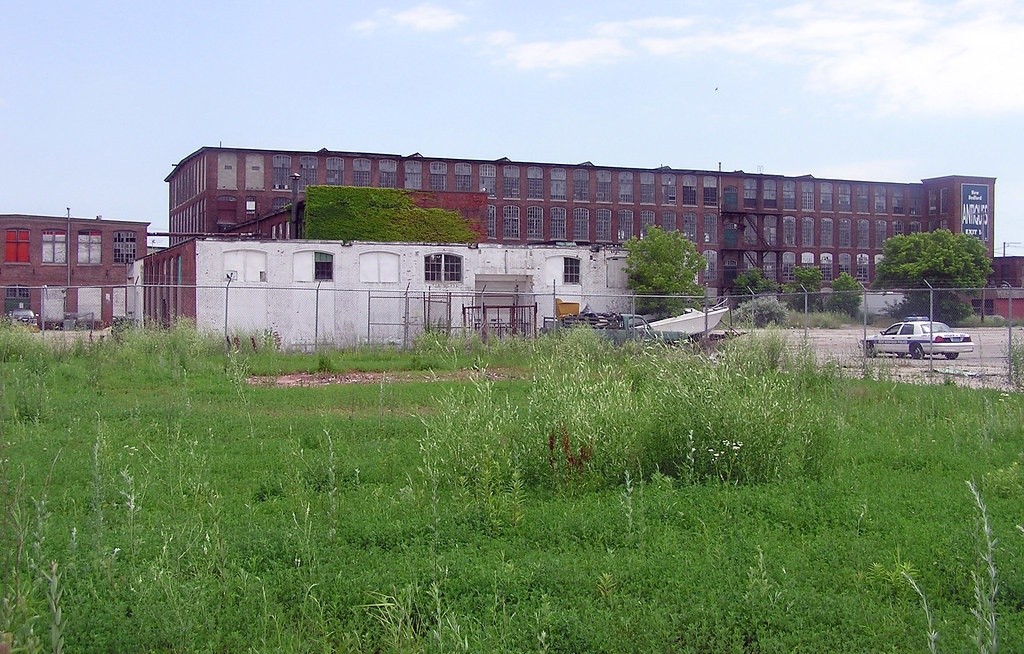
[541,313,689,347]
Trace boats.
[632,305,730,334]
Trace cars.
[5,309,40,326]
[861,315,974,360]
